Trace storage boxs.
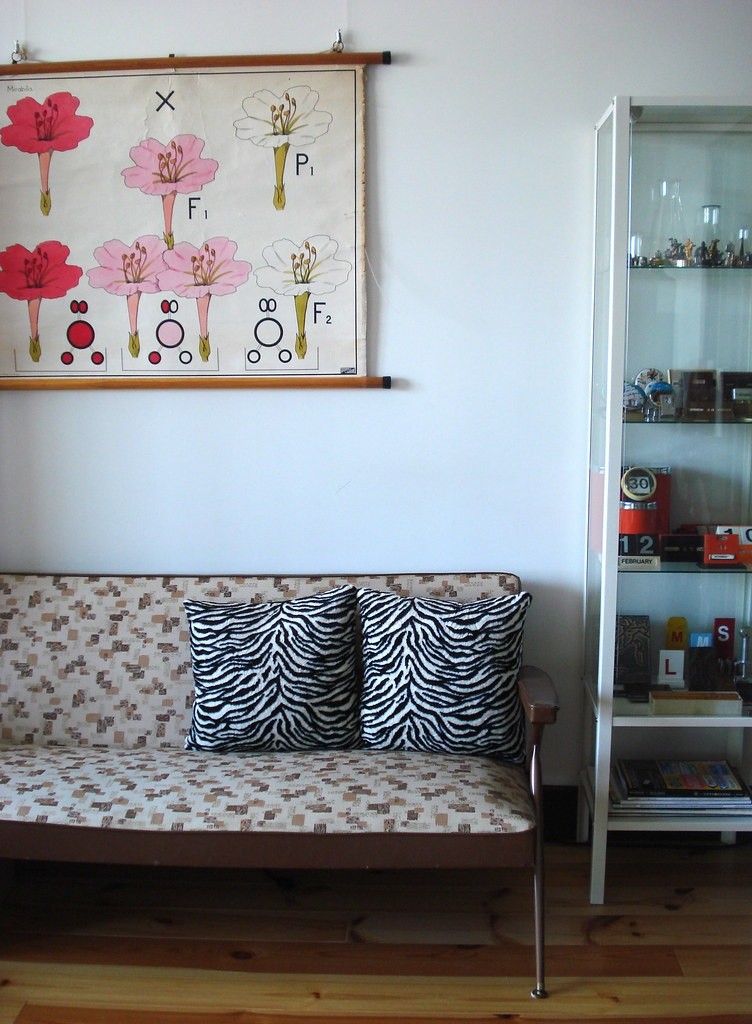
[649,691,744,716]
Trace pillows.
[355,587,534,765]
[182,584,364,755]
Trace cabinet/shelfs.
[577,93,752,907]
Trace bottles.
[650,180,689,268]
[702,205,724,267]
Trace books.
[606,757,752,817]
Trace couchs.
[0,574,560,1001]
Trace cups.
[620,502,657,534]
